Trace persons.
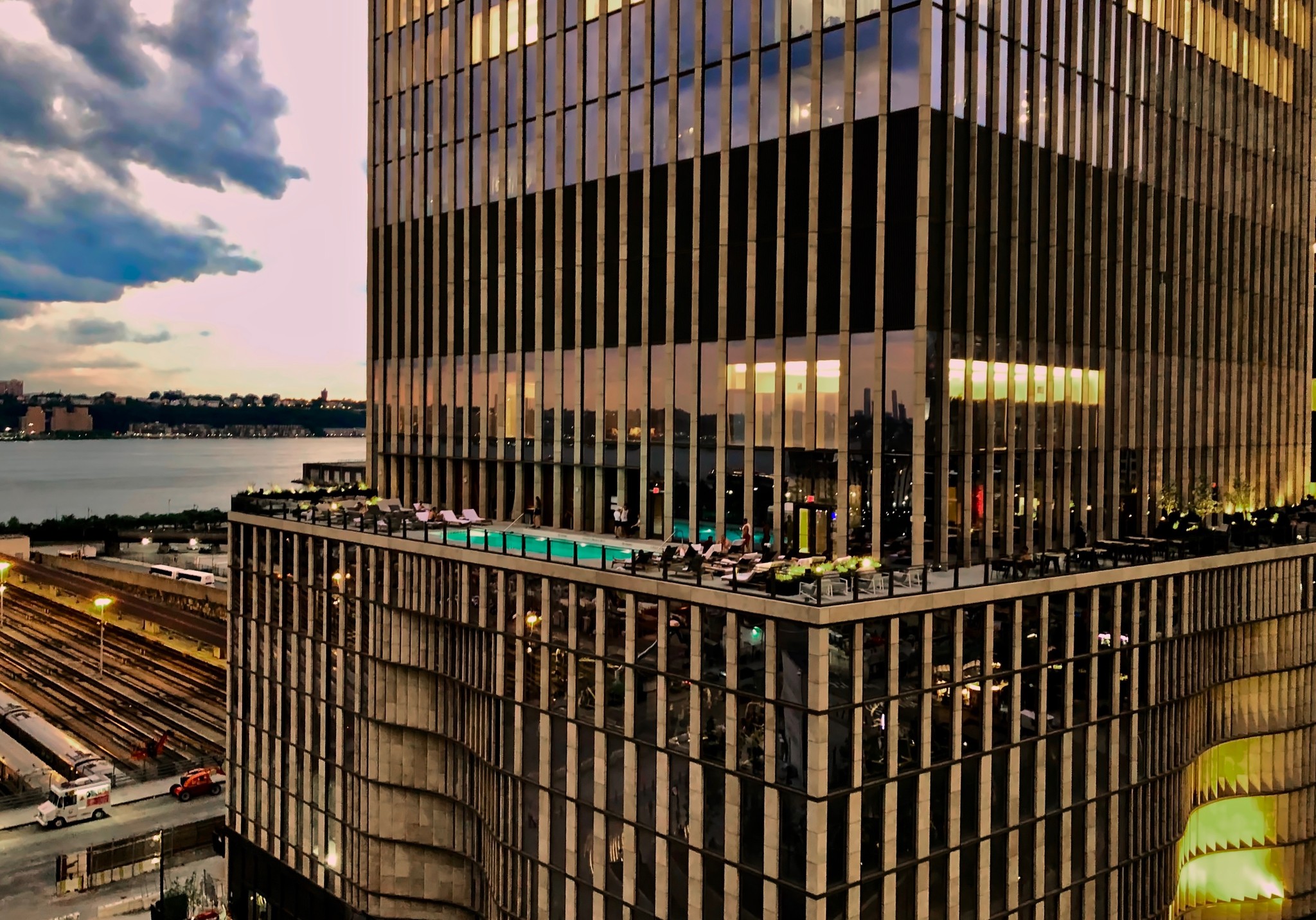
[612,506,640,539]
[740,518,751,556]
[1075,520,1087,548]
[784,515,794,546]
[427,506,438,522]
[613,535,730,562]
[417,501,425,511]
[533,496,542,529]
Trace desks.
[999,557,1023,579]
[1097,539,1114,561]
[1021,708,1054,732]
[1077,547,1106,569]
[1145,537,1166,559]
[1030,551,1066,575]
[1126,536,1145,544]
[1133,543,1152,564]
[1172,540,1188,559]
[1115,542,1133,561]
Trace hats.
[615,506,622,510]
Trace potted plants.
[766,556,882,596]
[606,682,651,734]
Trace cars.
[170,766,222,802]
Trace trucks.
[59,550,79,560]
[35,774,111,829]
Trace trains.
[0,690,136,788]
[0,728,68,798]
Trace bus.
[176,569,215,588]
[149,564,184,579]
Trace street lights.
[152,829,164,899]
[0,562,12,628]
[95,598,112,681]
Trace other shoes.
[537,526,540,528]
[615,536,619,538]
[531,525,535,528]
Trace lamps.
[507,441,513,446]
[527,441,534,446]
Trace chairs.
[990,504,1316,580]
[882,563,933,589]
[671,543,852,603]
[459,508,493,527]
[852,573,884,596]
[611,552,653,571]
[413,503,426,513]
[440,510,473,528]
[291,495,414,532]
[647,545,678,566]
[416,512,444,529]
[658,555,705,577]
